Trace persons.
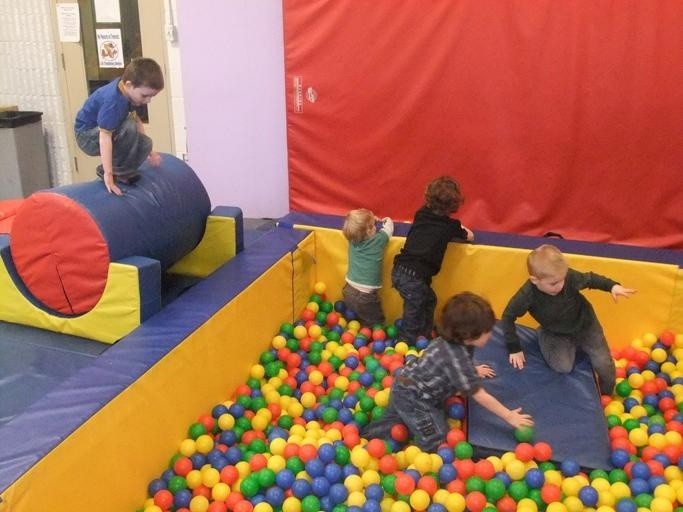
[501,245,637,393]
[74,58,165,196]
[343,207,394,328]
[359,292,535,454]
[391,176,474,342]
